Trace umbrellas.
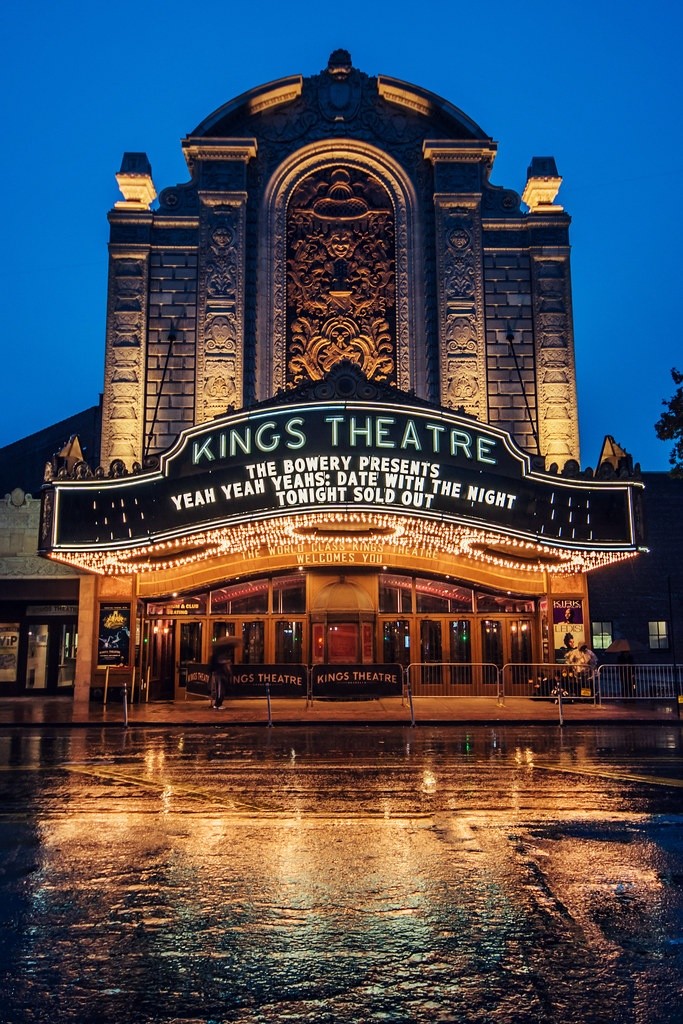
[212,636,244,666]
[604,639,649,654]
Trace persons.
[210,643,229,711]
[617,651,634,702]
